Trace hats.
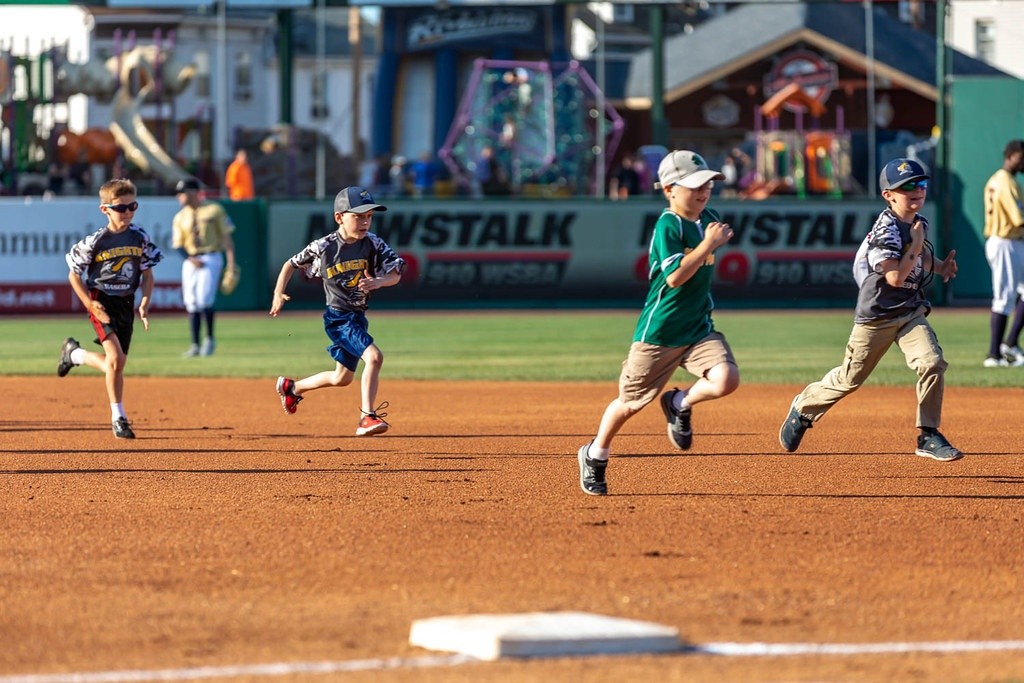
[167,178,202,197]
[879,158,932,192]
[654,149,726,190]
[334,186,388,213]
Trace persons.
[172,178,235,357]
[984,140,1024,369]
[57,180,164,439]
[611,157,646,197]
[269,187,404,437]
[577,150,740,495]
[780,158,962,463]
[224,151,257,201]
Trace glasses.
[102,201,139,213]
[900,179,928,190]
[691,180,715,190]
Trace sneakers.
[276,376,304,415]
[356,401,392,436]
[779,394,813,452]
[660,387,693,450]
[58,337,81,377]
[112,416,136,440]
[577,438,609,496]
[914,432,964,462]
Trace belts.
[195,251,216,256]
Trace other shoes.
[983,343,1024,367]
[185,342,200,356]
[201,337,215,356]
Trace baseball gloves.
[220,265,240,294]
